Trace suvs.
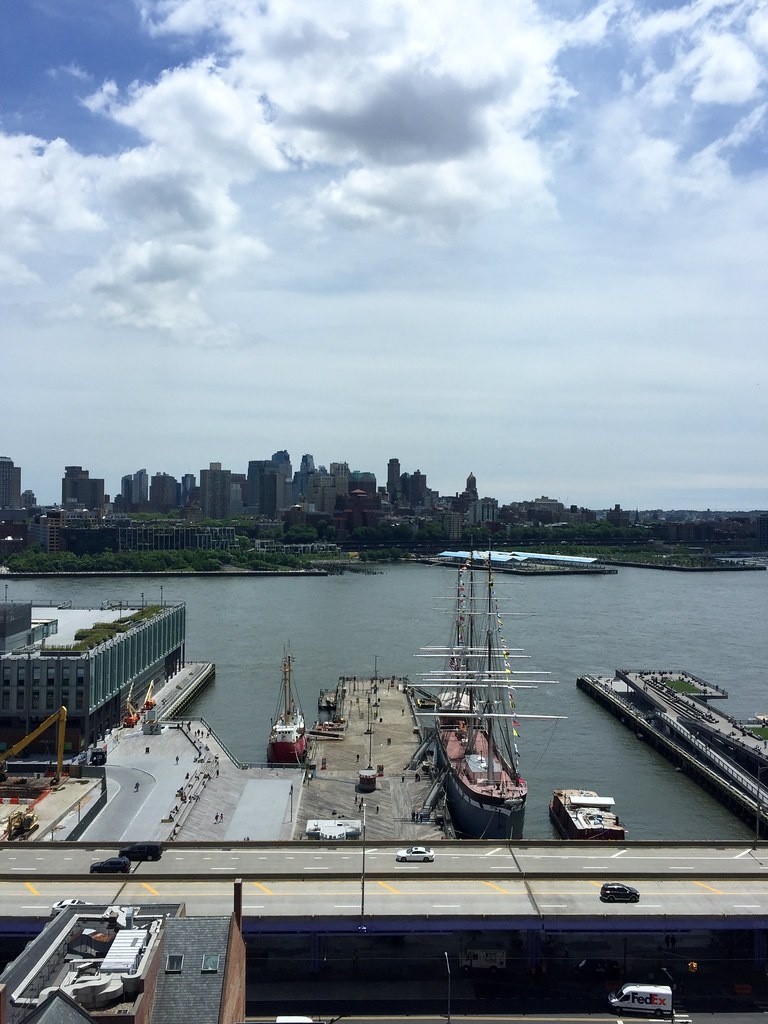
[118,841,162,861]
[90,857,132,874]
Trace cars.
[397,846,435,863]
[600,882,640,903]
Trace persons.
[355,793,379,814]
[134,721,225,824]
[353,675,421,783]
[411,810,423,824]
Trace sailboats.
[402,536,570,839]
[265,640,310,766]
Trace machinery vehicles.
[7,811,40,841]
[142,680,157,710]
[0,705,68,785]
[123,681,140,729]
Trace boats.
[549,788,628,841]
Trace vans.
[606,984,673,1019]
